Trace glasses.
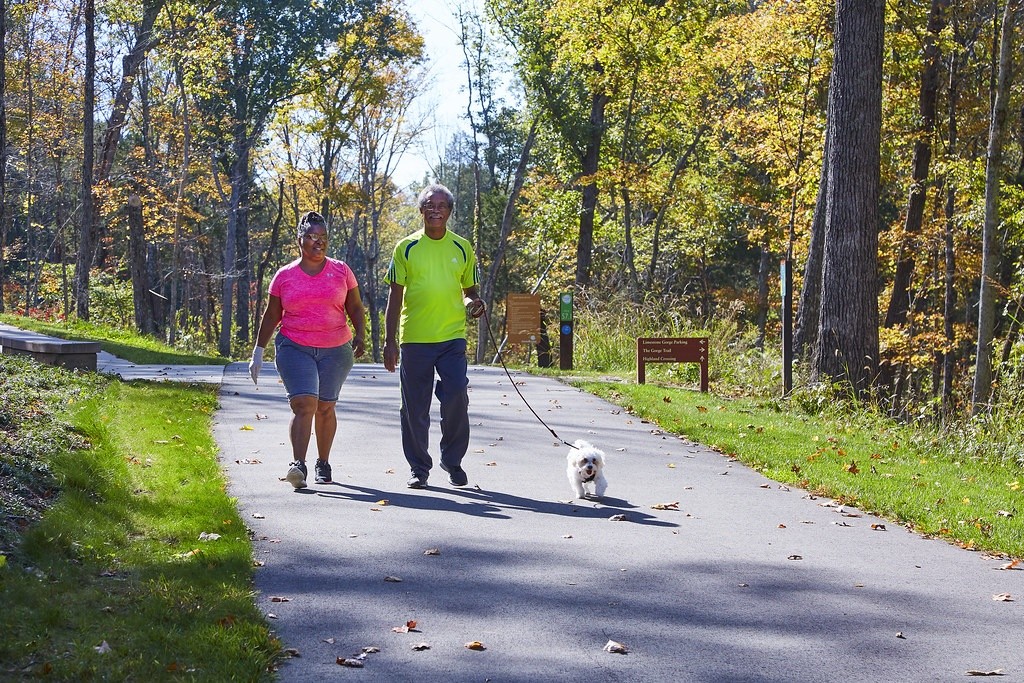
[304,232,331,243]
[423,202,450,212]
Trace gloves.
[248,346,264,385]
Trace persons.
[249,211,366,488]
[382,185,488,488]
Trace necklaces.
[301,258,327,276]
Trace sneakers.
[406,474,428,488]
[440,457,468,486]
[314,459,332,484]
[286,459,308,489]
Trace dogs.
[564,438,608,499]
